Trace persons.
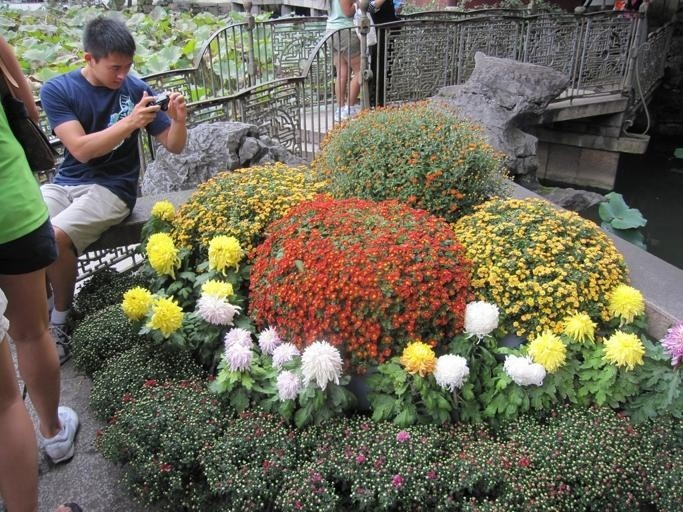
[0,288,80,512]
[0,34,79,463]
[366,0,401,108]
[326,0,361,122]
[40,16,186,368]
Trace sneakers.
[335,109,361,122]
[39,406,78,462]
[49,323,69,362]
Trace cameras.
[146,95,170,111]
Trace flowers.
[450,196,631,343]
[250,198,475,378]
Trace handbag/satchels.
[5,94,60,173]
[354,10,377,47]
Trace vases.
[490,329,529,361]
[346,364,387,412]
